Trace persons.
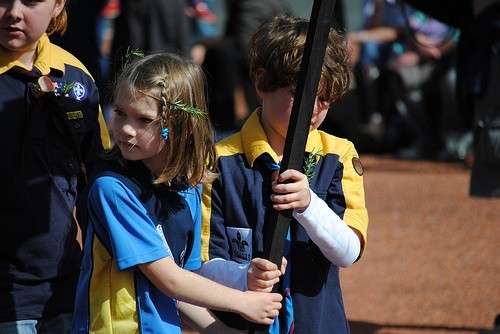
[69,53,283,334]
[201,12,370,334]
[46,0,500,161]
[0,0,113,334]
